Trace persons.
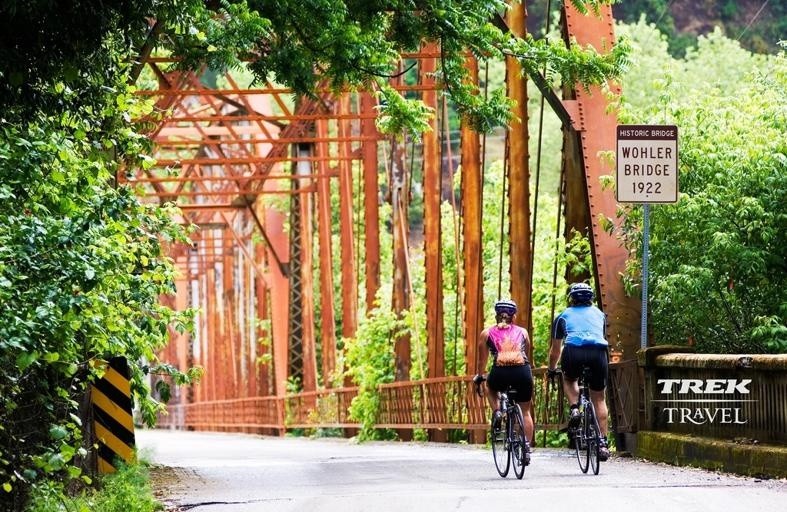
[473,298,535,466]
[543,283,610,461]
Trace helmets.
[566,282,594,301]
[495,301,518,316]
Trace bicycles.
[547,371,606,475]
[476,379,528,479]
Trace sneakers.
[599,447,611,459]
[490,409,503,437]
[522,452,531,464]
[568,408,581,429]
